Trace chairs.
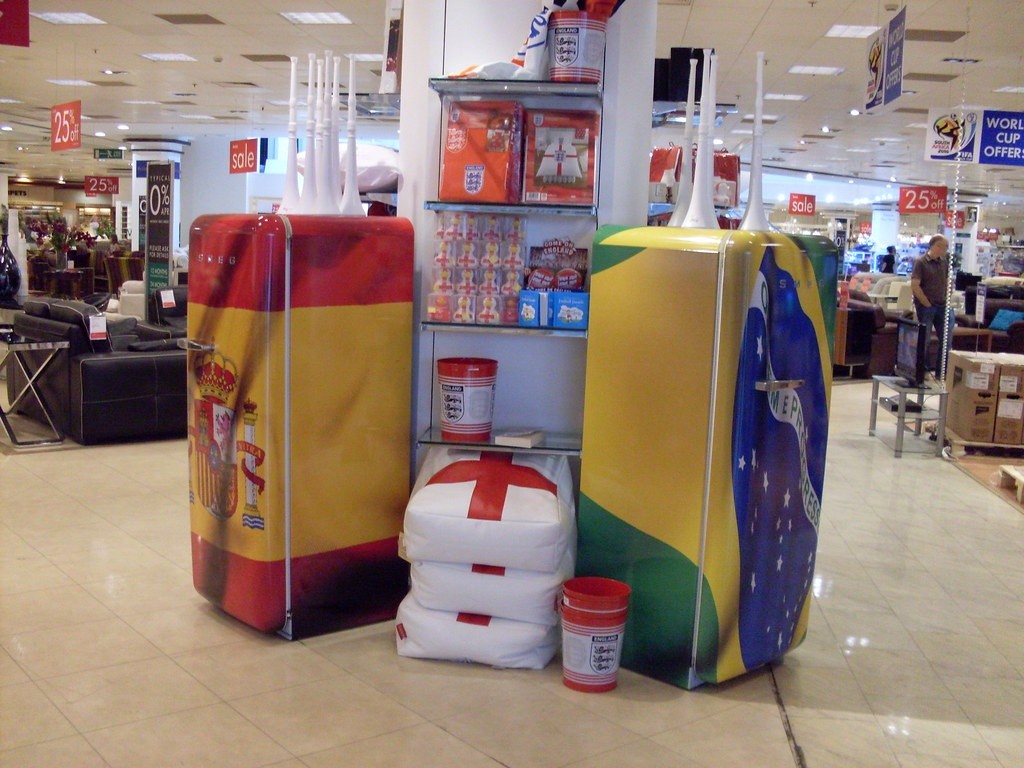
[26,247,144,300]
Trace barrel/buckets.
[557,577,632,693]
[436,357,499,442]
[547,10,608,83]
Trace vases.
[55,250,67,270]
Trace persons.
[107,234,121,257]
[886,246,901,273]
[880,247,895,273]
[910,234,955,381]
[85,242,95,266]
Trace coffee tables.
[932,328,993,352]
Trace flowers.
[28,207,98,261]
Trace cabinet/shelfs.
[415,78,602,456]
[834,308,873,379]
[869,374,949,459]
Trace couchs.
[137,285,189,343]
[839,271,1024,353]
[836,290,899,381]
[6,298,187,445]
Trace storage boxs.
[944,349,1002,444]
[980,352,1024,445]
